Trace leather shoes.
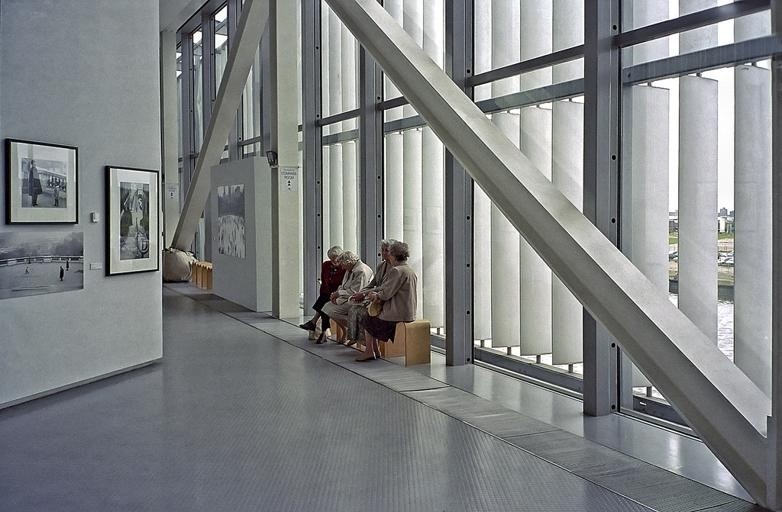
[356,351,381,361]
[337,336,355,346]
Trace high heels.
[317,333,327,344]
[300,321,315,331]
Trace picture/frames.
[6,137,82,225]
[103,164,162,274]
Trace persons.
[58,265,64,281]
[53,185,59,206]
[355,242,417,361]
[346,239,399,347]
[66,258,70,271]
[28,159,42,208]
[321,251,375,347]
[300,246,344,343]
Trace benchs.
[323,317,432,367]
